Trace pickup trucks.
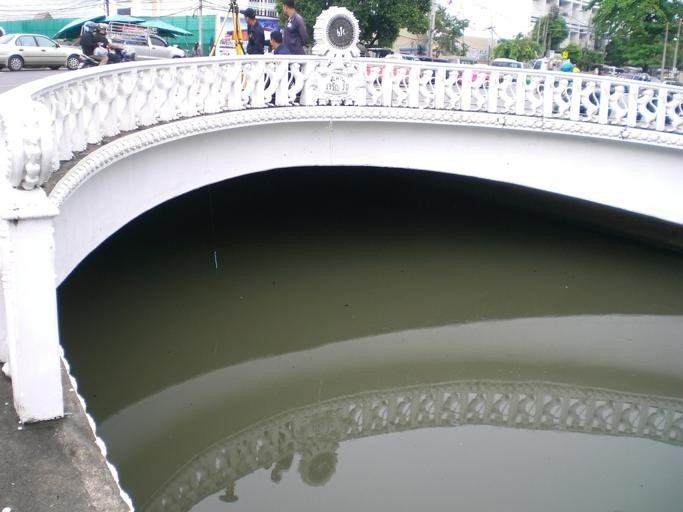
[107,31,185,62]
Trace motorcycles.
[74,41,135,71]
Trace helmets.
[84,21,109,35]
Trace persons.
[193,42,202,57]
[93,23,125,64]
[80,21,109,66]
[264,32,292,106]
[281,0,310,105]
[240,8,265,109]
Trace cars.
[0,32,85,73]
[589,63,683,94]
[365,47,500,88]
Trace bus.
[491,58,533,86]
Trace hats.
[240,8,256,18]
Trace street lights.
[531,15,540,45]
[488,26,495,46]
[672,37,679,68]
[530,20,536,23]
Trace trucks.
[528,57,570,82]
